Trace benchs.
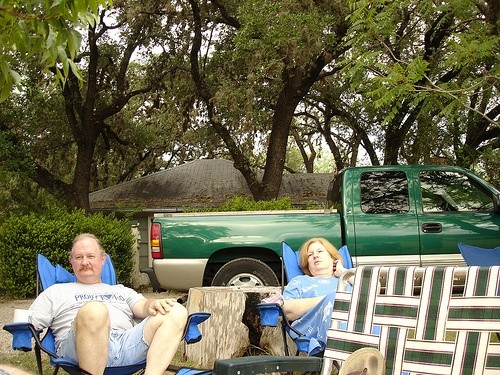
[214,265,500,375]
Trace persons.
[281,237,409,375]
[28,233,189,375]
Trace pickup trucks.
[148,165,500,290]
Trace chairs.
[3,254,211,375]
[457,242,500,266]
[255,241,354,375]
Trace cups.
[13,309,28,322]
[261,290,285,307]
